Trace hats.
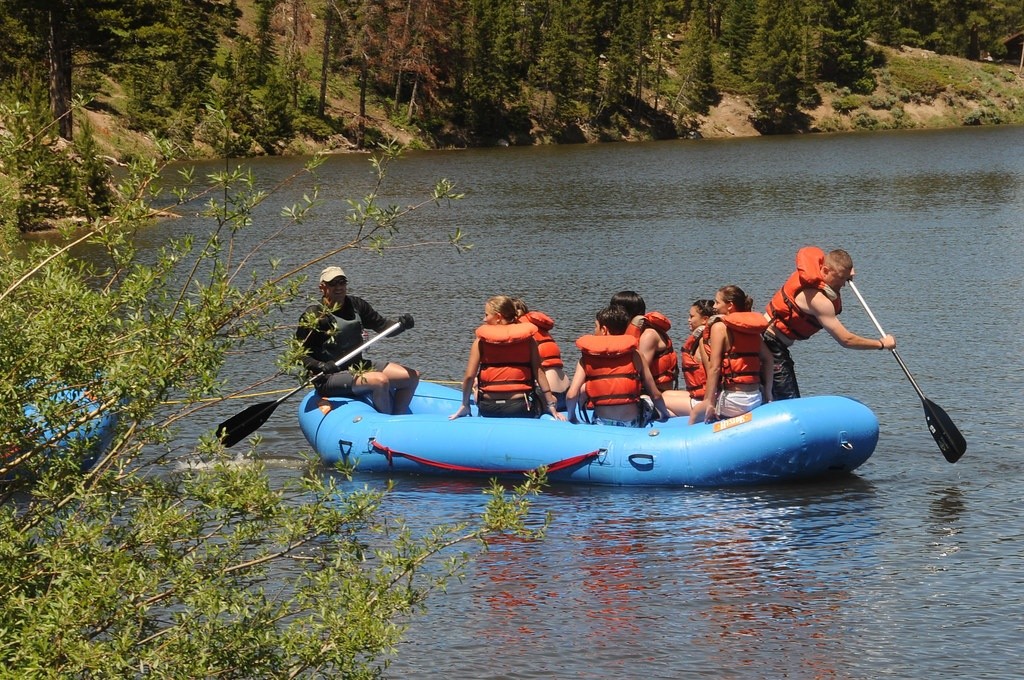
[320,267,346,284]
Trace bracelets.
[879,340,884,350]
[544,390,550,393]
[546,402,555,407]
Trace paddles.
[845,274,968,464]
[215,313,416,452]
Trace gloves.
[322,361,340,373]
[400,313,415,329]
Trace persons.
[611,291,677,393]
[662,285,776,425]
[761,246,896,399]
[296,267,420,414]
[448,296,566,420]
[510,297,570,412]
[566,305,676,428]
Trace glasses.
[326,279,346,287]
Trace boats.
[0,367,132,484]
[297,380,880,489]
